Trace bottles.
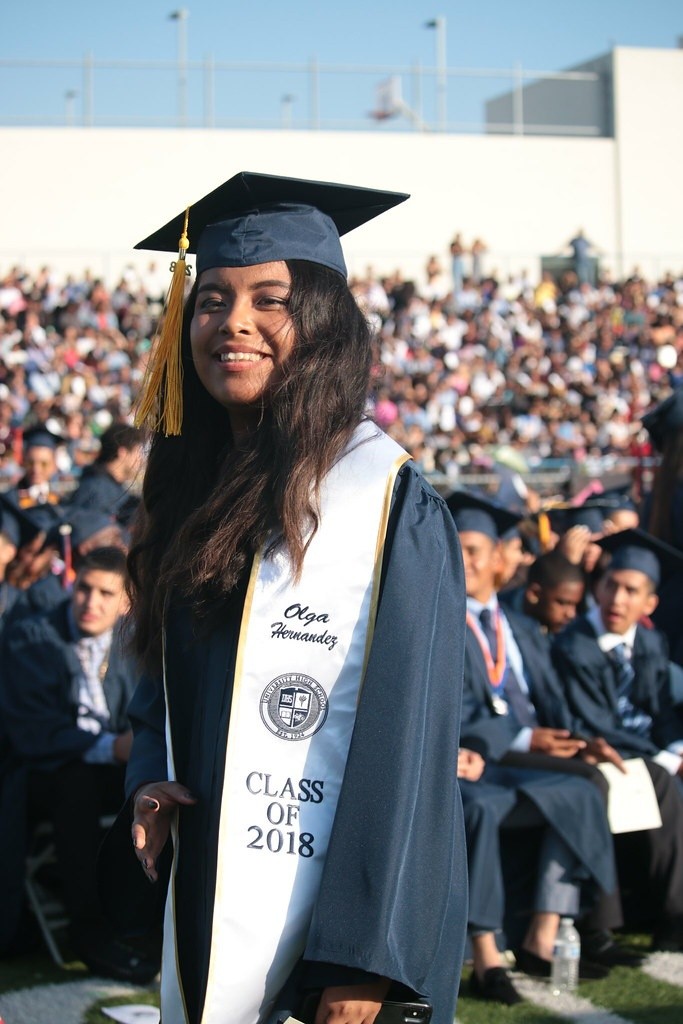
[549,918,581,998]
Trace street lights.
[169,8,189,126]
[425,15,450,128]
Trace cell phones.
[301,994,434,1024]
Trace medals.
[490,690,511,715]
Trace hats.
[591,528,683,588]
[499,527,520,544]
[21,503,61,535]
[22,422,66,454]
[547,506,604,537]
[134,171,410,438]
[585,483,641,522]
[56,508,123,559]
[446,489,527,548]
[0,493,42,549]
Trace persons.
[126,171,469,1023]
[0,233,683,1001]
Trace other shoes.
[67,905,157,982]
[590,939,644,968]
[516,947,551,977]
[470,968,520,1005]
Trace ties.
[479,609,536,727]
[614,644,634,710]
